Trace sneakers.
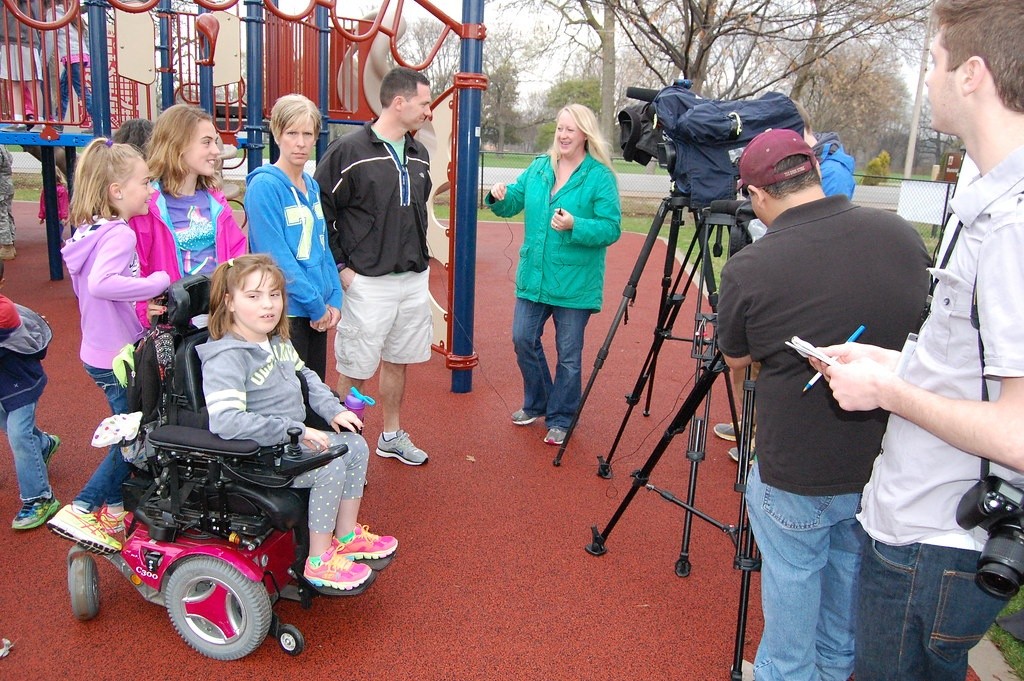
[42,435,61,466]
[376,429,429,466]
[47,504,123,555]
[82,123,94,133]
[714,421,755,442]
[731,659,757,681]
[303,546,372,591]
[332,523,398,561]
[729,438,755,461]
[0,247,17,259]
[544,429,566,444]
[100,510,130,533]
[60,240,66,249]
[12,491,60,529]
[511,409,538,425]
[53,125,64,133]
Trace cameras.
[956,476,1024,602]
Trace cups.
[343,393,366,435]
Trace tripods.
[552,185,761,681]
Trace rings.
[555,224,557,228]
[492,191,495,194]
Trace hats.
[736,128,817,191]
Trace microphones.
[401,163,408,167]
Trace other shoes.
[26,115,36,131]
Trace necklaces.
[300,179,303,188]
[318,327,325,331]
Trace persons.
[46,138,170,557]
[196,254,397,590]
[112,104,247,329]
[485,104,621,444]
[313,68,434,466]
[0,0,95,529]
[781,101,891,201]
[715,130,933,681]
[245,93,343,384]
[789,0,1024,681]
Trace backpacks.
[118,314,193,480]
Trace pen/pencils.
[803,326,865,392]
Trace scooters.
[66,275,400,661]
